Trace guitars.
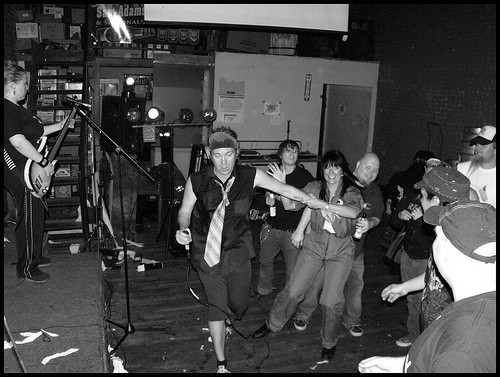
[24,96,81,198]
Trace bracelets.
[325,203,329,210]
[36,155,49,168]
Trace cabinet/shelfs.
[30,57,89,251]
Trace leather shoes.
[321,344,336,361]
[252,323,272,340]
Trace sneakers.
[38,256,51,266]
[17,266,50,284]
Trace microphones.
[61,95,92,108]
[182,231,192,260]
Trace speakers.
[100,95,147,155]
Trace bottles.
[268,191,276,216]
[352,213,366,242]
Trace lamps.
[146,106,166,125]
[178,107,194,124]
[198,107,217,123]
[123,76,136,92]
[125,107,145,127]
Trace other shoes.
[349,325,363,337]
[396,335,412,347]
[294,320,308,330]
[216,365,231,373]
[249,291,264,302]
[208,325,231,343]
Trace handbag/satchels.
[384,231,405,266]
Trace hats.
[423,200,496,263]
[413,166,471,202]
[468,125,496,145]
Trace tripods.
[77,109,171,355]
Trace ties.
[203,176,235,267]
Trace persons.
[248,139,317,299]
[293,153,383,336]
[389,158,442,347]
[381,166,471,333]
[358,200,496,373]
[456,125,496,210]
[3,59,75,283]
[175,126,311,373]
[251,150,363,361]
